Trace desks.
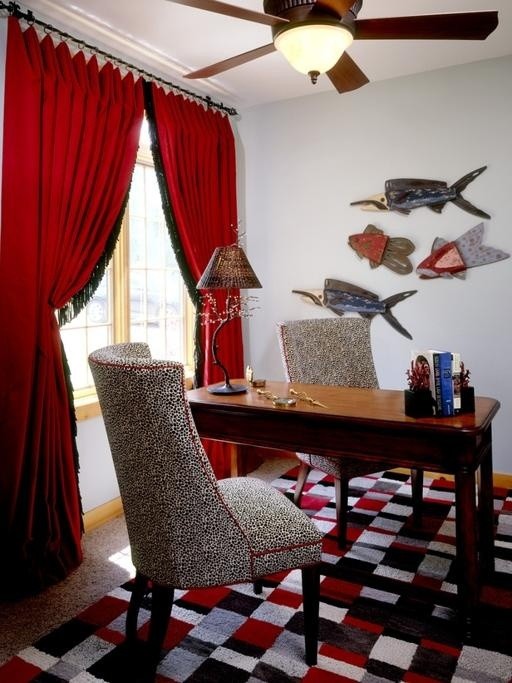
[184,376,501,607]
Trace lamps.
[270,23,356,85]
[191,247,263,396]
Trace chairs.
[88,341,322,667]
[275,316,425,548]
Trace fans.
[170,0,500,94]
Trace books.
[410,347,442,417]
[451,351,463,414]
[425,349,454,417]
